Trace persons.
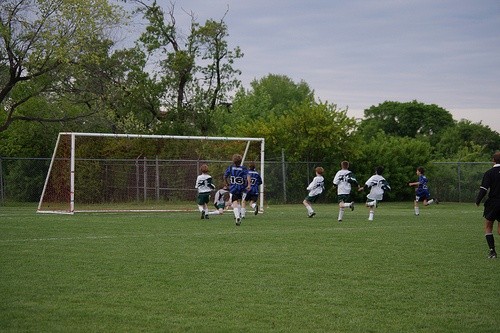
[223,153,251,226]
[208,184,232,215]
[240,162,262,220]
[195,164,212,220]
[408,166,440,216]
[476,152,500,259]
[302,167,325,218]
[332,160,360,221]
[358,166,392,221]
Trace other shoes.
[254,204,259,215]
[350,201,354,211]
[236,217,240,226]
[433,197,439,204]
[338,218,342,221]
[201,210,205,219]
[414,214,419,216]
[488,252,497,258]
[307,210,316,218]
[373,199,378,208]
[241,215,245,219]
[205,215,209,219]
